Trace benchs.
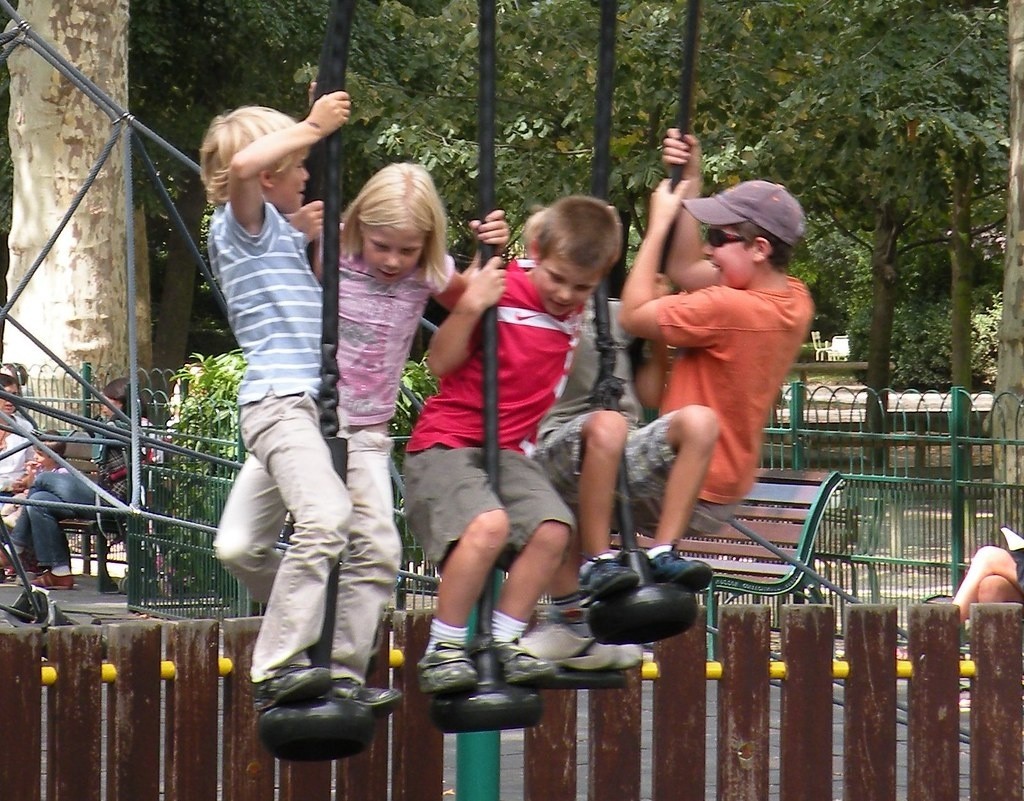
[59,442,127,593]
[609,467,845,661]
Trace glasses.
[704,227,751,248]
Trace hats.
[680,180,806,247]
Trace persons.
[199,82,814,712]
[0,363,216,590]
[951,546,1024,623]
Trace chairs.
[809,331,850,361]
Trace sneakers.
[567,624,645,669]
[415,646,479,694]
[251,665,331,713]
[576,548,641,609]
[331,678,404,720]
[649,552,713,593]
[495,639,559,685]
[28,570,74,590]
